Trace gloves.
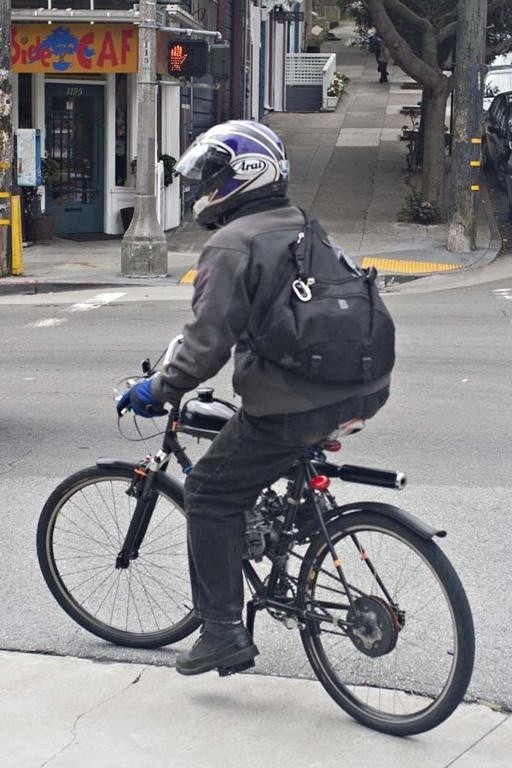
[116,376,168,422]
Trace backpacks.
[245,199,397,384]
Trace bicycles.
[35,335,476,737]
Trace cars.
[480,56,512,221]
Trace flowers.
[327,72,351,97]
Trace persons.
[114,118,398,678]
[375,37,389,83]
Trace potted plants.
[25,156,64,241]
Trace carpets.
[55,232,124,242]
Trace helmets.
[174,119,290,225]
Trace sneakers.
[276,484,328,528]
[175,619,260,676]
